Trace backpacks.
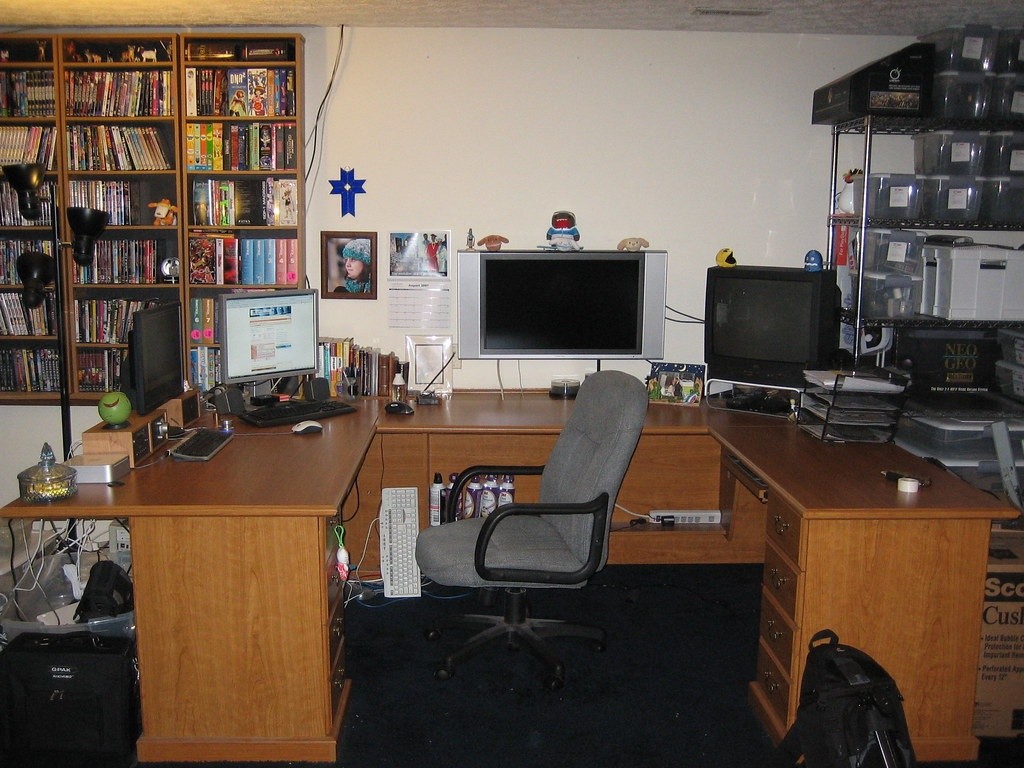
[73,560,134,624]
[772,629,918,768]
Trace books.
[69,180,132,225]
[193,178,298,226]
[189,229,298,284]
[186,67,296,117]
[0,181,60,392]
[190,289,275,391]
[73,237,166,284]
[73,299,169,393]
[0,70,174,171]
[187,122,297,171]
[298,337,409,397]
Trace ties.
[433,244,434,247]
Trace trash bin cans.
[3,630,135,768]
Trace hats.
[343,239,370,265]
[431,234,437,237]
[436,238,442,241]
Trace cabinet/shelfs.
[0,394,390,762]
[709,426,1022,766]
[827,114,1024,420]
[342,392,793,581]
[796,361,913,443]
[0,32,306,405]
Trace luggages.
[0,631,139,768]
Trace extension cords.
[647,510,721,523]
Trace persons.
[421,233,447,275]
[466,229,475,248]
[166,260,178,275]
[334,240,370,292]
[661,373,683,397]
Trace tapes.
[896,478,919,493]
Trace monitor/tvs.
[119,301,185,416]
[704,265,842,389]
[457,250,668,361]
[219,289,319,410]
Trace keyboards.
[236,401,356,429]
[164,429,235,461]
[380,487,422,598]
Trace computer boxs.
[108,519,133,583]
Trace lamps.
[0,162,111,553]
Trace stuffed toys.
[546,210,581,247]
[477,235,509,250]
[617,238,650,251]
[148,198,178,225]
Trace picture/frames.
[647,363,705,406]
[321,231,377,300]
[406,335,452,394]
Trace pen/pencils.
[880,470,932,487]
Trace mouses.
[385,401,415,415]
[291,420,323,434]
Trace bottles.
[481,474,501,519]
[463,474,483,520]
[447,473,463,524]
[430,471,446,526]
[499,474,515,508]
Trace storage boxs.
[0,518,135,643]
[810,22,1024,527]
[159,389,201,427]
[61,452,130,483]
[973,532,1024,738]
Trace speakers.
[303,377,329,402]
[215,388,247,415]
